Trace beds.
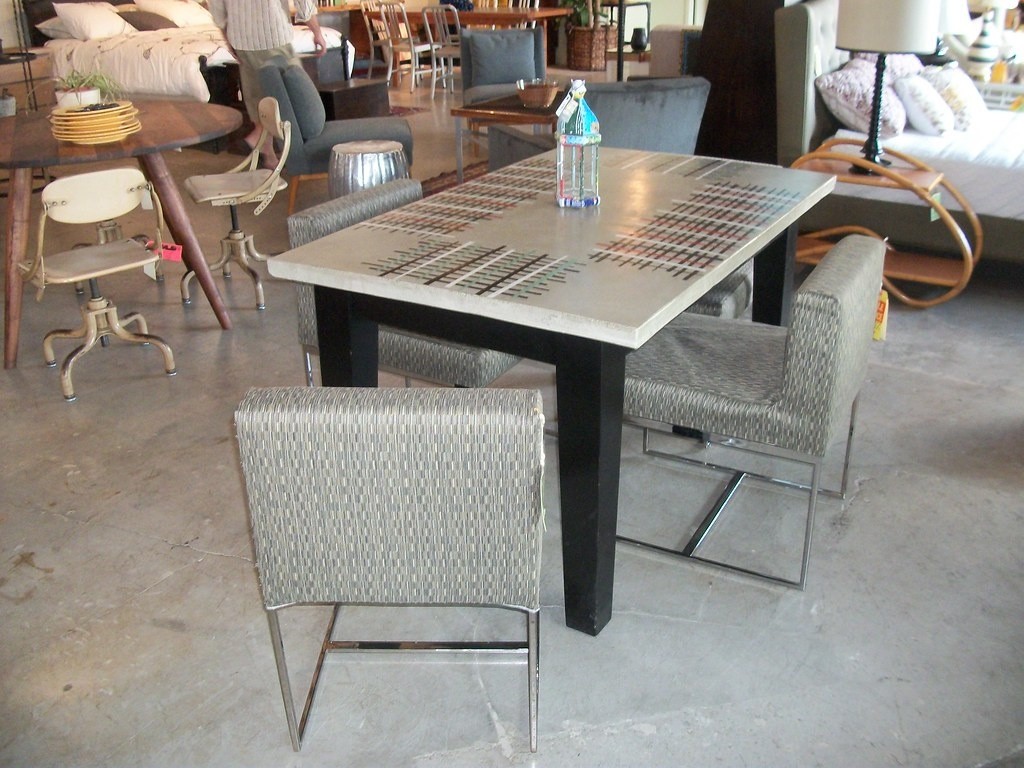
[20,0,356,154]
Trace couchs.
[779,2,1024,262]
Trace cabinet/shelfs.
[793,137,983,307]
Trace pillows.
[925,62,988,128]
[468,33,539,84]
[816,57,905,135]
[35,0,212,42]
[895,75,957,133]
[854,51,925,82]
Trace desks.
[365,6,574,87]
[0,98,243,369]
[266,145,840,635]
[448,90,564,191]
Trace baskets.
[518,85,558,107]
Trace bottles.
[554,80,602,209]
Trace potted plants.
[24,68,135,114]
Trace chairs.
[179,96,292,311]
[17,165,176,401]
[598,0,652,45]
[488,77,712,174]
[614,236,887,590]
[231,386,545,753]
[459,26,549,154]
[359,0,541,99]
[286,180,522,387]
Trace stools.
[328,139,411,198]
[314,75,390,121]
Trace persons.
[206,0,326,180]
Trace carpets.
[388,104,433,116]
[419,159,491,198]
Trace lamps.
[835,1,940,172]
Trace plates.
[49,101,142,145]
